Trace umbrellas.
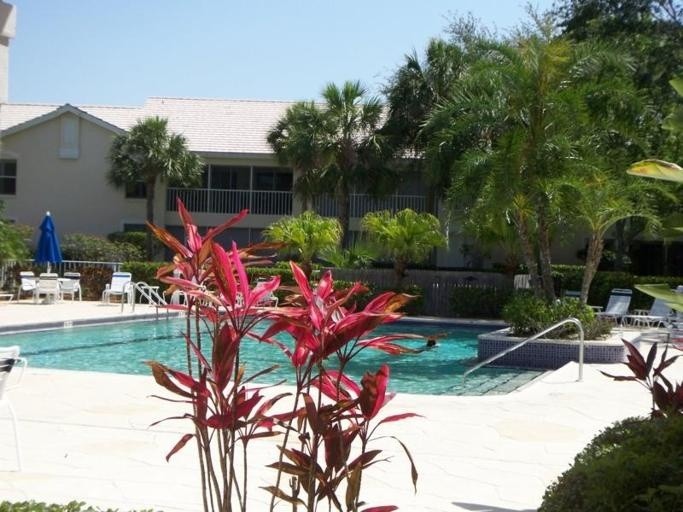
[36,211,63,302]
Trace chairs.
[0,346,27,398]
[0,267,279,313]
[564,282,682,348]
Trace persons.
[416,340,438,353]
